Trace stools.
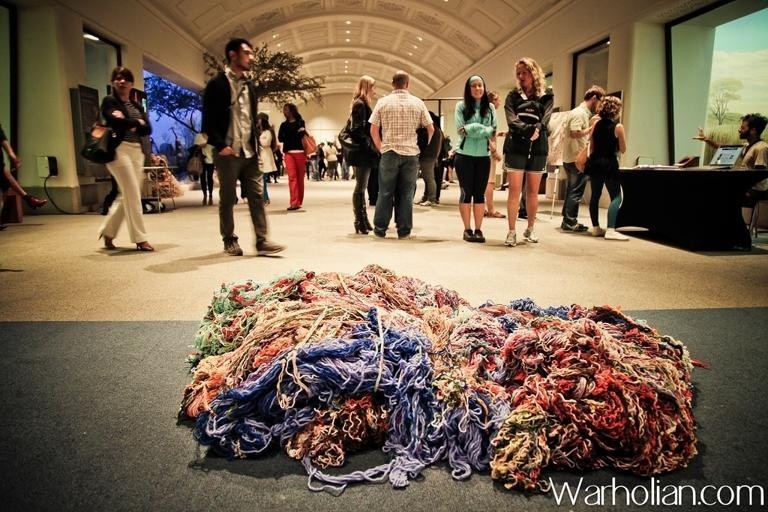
[747,199,768,239]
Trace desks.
[618,167,768,252]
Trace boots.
[353,193,373,234]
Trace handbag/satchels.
[339,119,370,148]
[81,124,117,164]
[302,135,316,155]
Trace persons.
[502,57,554,248]
[370,71,436,241]
[0,124,48,231]
[193,103,355,209]
[692,116,768,206]
[349,75,376,234]
[201,37,287,255]
[483,90,505,219]
[367,109,454,207]
[97,66,154,251]
[590,97,631,242]
[559,85,605,233]
[454,72,497,247]
[517,166,539,218]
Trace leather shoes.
[28,193,49,210]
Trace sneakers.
[591,227,606,237]
[223,242,243,256]
[420,200,436,206]
[449,181,457,184]
[462,208,539,245]
[275,181,278,183]
[414,200,426,205]
[203,199,207,205]
[561,222,588,231]
[265,201,270,207]
[374,227,386,237]
[441,181,449,189]
[605,231,629,240]
[266,180,272,182]
[397,230,410,238]
[287,207,302,211]
[319,175,356,181]
[208,199,213,206]
[256,239,288,255]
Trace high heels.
[136,241,154,251]
[98,234,119,250]
